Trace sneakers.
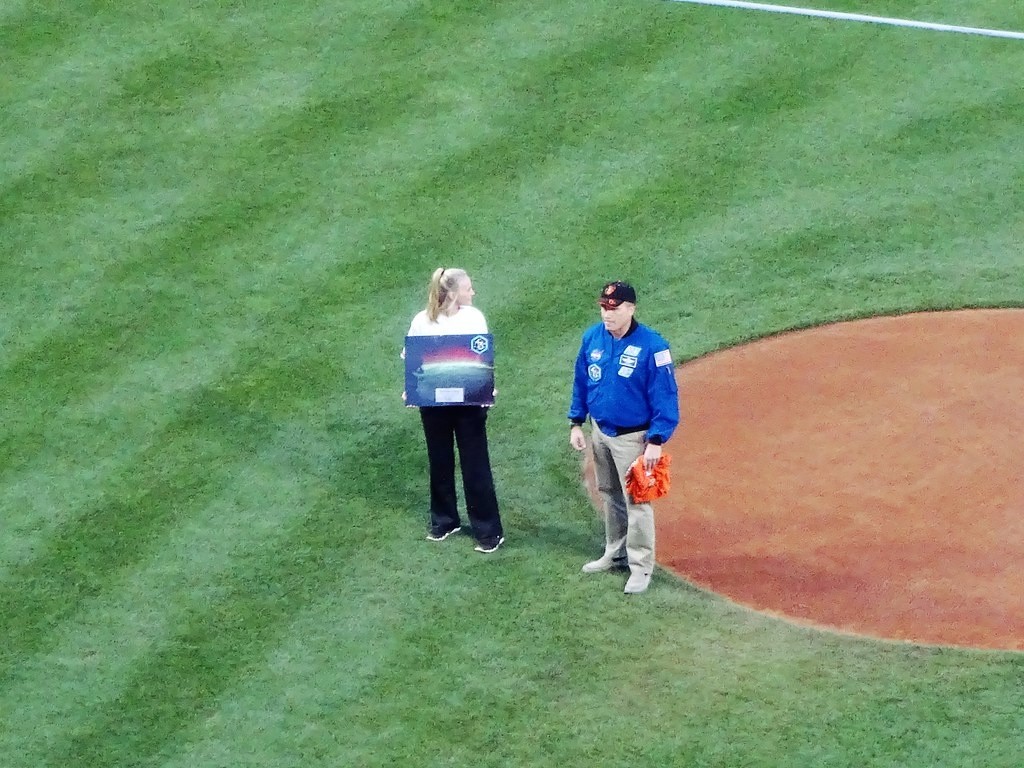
[474,536,504,553]
[624,572,650,594]
[426,523,461,542]
[583,553,629,574]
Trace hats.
[599,281,636,306]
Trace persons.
[400,267,504,554]
[568,282,680,595]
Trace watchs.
[569,422,580,429]
